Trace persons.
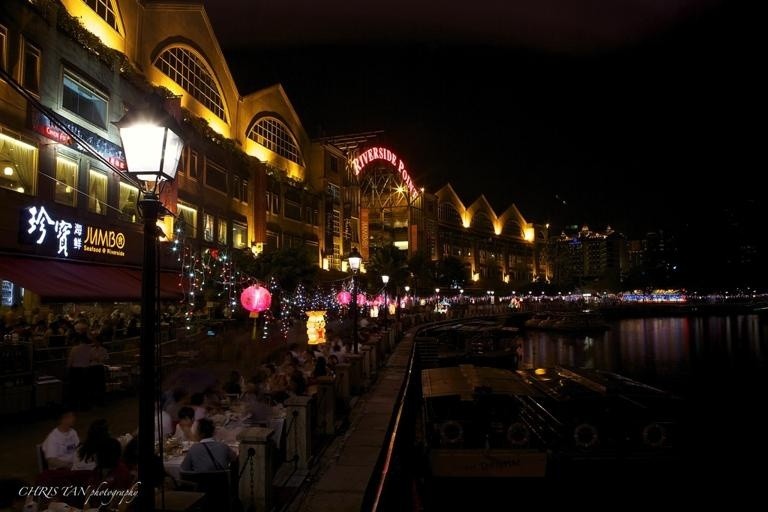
[5,306,430,511]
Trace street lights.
[381,274,391,331]
[346,246,368,355]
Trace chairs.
[5,437,240,511]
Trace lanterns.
[354,293,367,306]
[337,290,351,305]
[239,283,273,319]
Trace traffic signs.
[110,107,187,512]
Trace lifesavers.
[440,421,464,444]
[574,423,598,448]
[642,424,666,448]
[507,422,530,446]
